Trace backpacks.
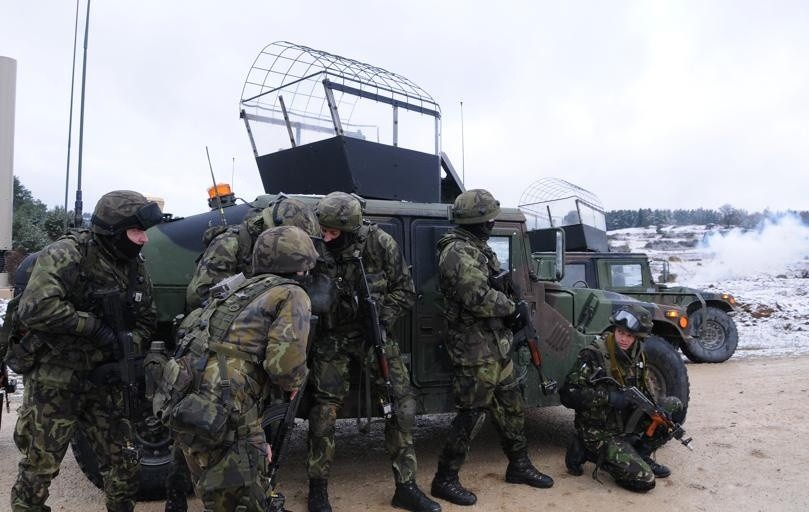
[152,355,193,426]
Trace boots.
[166,487,189,512]
[642,455,670,478]
[308,444,586,512]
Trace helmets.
[252,192,366,273]
[608,304,653,338]
[453,190,500,224]
[90,191,153,238]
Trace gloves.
[606,392,632,410]
[114,331,133,358]
[364,322,387,351]
[81,315,123,361]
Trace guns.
[262,314,319,480]
[349,257,393,404]
[91,284,140,420]
[490,268,548,396]
[590,367,695,452]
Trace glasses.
[614,308,641,331]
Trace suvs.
[72,40,690,500]
[518,176,738,362]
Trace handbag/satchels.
[169,351,232,442]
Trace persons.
[559,305,684,491]
[7,188,163,512]
[303,191,442,511]
[431,188,553,507]
[176,226,320,511]
[164,199,319,512]
